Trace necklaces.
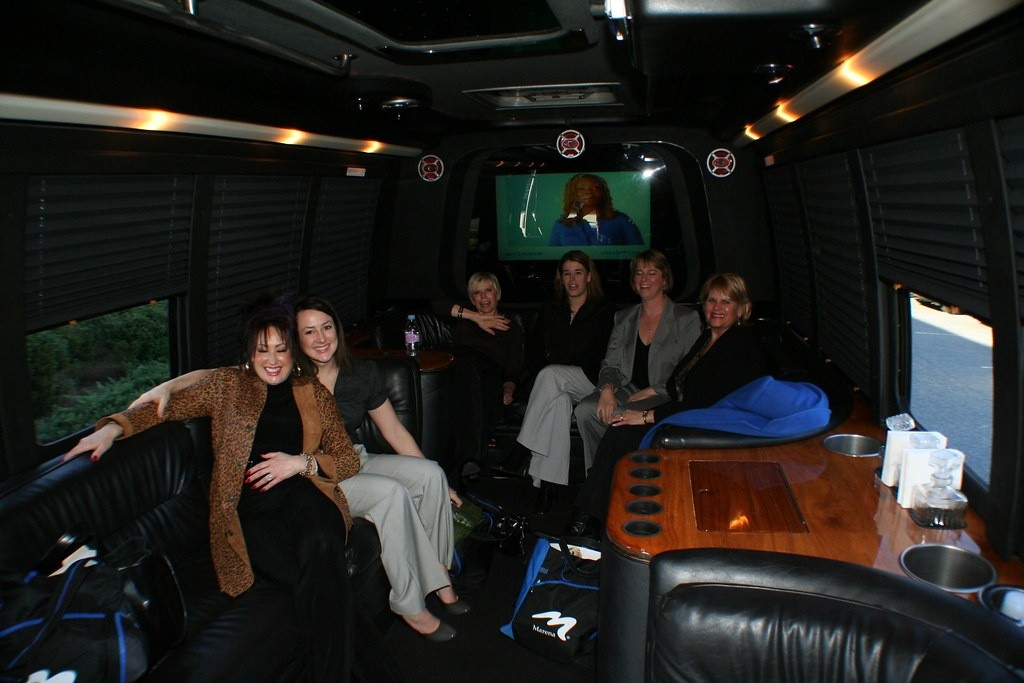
[647,328,652,332]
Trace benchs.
[650,304,855,450]
[373,304,585,477]
[0,357,422,682]
[644,548,1024,683]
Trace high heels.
[491,450,532,478]
[431,589,471,616]
[400,609,458,643]
[533,486,559,518]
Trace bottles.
[911,452,968,529]
[404,314,421,357]
[878,412,916,476]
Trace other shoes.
[561,513,602,541]
[461,462,481,481]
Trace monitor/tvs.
[493,170,653,261]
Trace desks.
[355,347,455,483]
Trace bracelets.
[643,410,649,424]
[458,306,464,319]
[300,452,312,476]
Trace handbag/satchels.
[452,495,484,547]
[469,492,527,557]
[500,536,605,672]
[0,522,188,683]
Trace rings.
[266,474,273,481]
[620,416,623,422]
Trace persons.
[549,173,644,247]
[585,273,780,539]
[127,304,472,642]
[574,251,704,479]
[62,301,404,682]
[515,250,620,494]
[427,274,527,489]
